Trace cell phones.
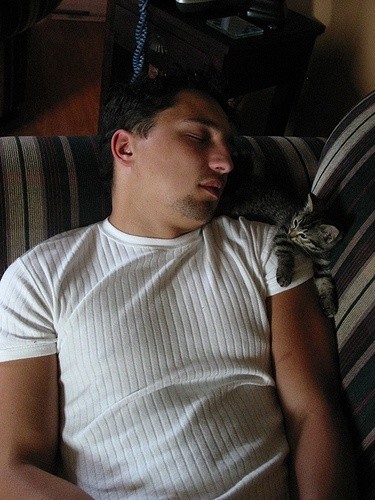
[206,16,264,39]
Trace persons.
[0,80,356,500]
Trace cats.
[222,190,346,319]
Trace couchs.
[0,92,375,500]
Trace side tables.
[98,0,326,136]
